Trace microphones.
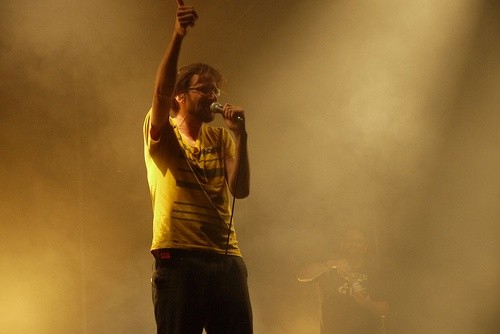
[209,102,245,123]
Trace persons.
[142,0,254,334]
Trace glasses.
[184,85,223,97]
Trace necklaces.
[175,117,204,156]
